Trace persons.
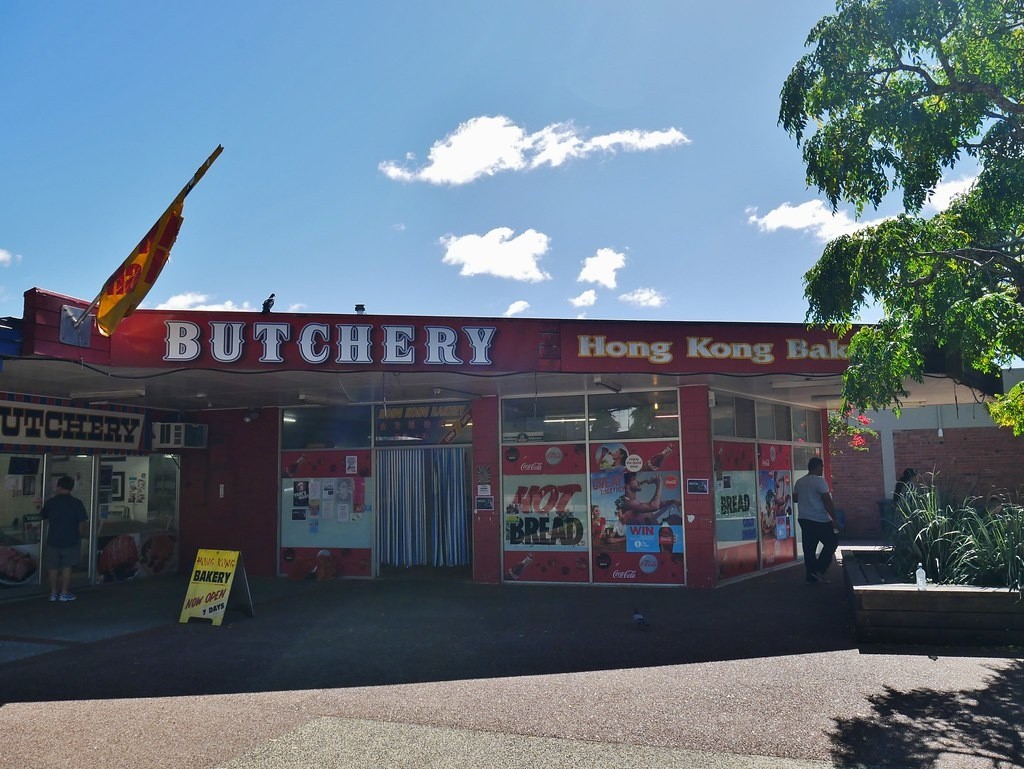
[625,474,682,525]
[892,469,918,534]
[591,504,606,542]
[760,512,778,536]
[606,507,629,542]
[794,457,840,584]
[598,446,627,467]
[40,477,88,601]
[771,472,791,516]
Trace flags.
[97,187,184,338]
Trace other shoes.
[49,593,57,602]
[811,571,831,585]
[805,579,812,585]
[58,592,77,602]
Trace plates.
[0,554,39,586]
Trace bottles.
[603,447,612,456]
[642,443,673,471]
[639,477,663,485]
[786,511,790,539]
[658,518,674,552]
[284,454,306,478]
[606,528,610,543]
[714,447,724,471]
[504,554,535,580]
